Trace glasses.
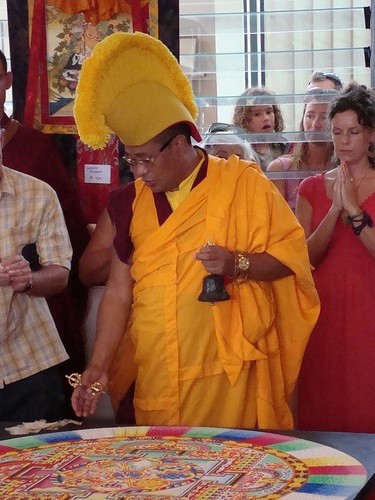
[208,122,246,140]
[65,373,106,396]
[122,133,180,167]
[306,87,338,100]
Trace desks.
[0,421,375,500]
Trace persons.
[203,70,375,212]
[293,82,375,434]
[71,31,322,432]
[1,48,136,421]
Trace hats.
[73,30,202,151]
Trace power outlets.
[84,164,111,184]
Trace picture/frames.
[28,1,159,134]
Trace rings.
[350,178,353,182]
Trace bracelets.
[18,283,32,293]
[228,250,251,283]
[348,211,373,236]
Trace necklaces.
[351,174,366,192]
